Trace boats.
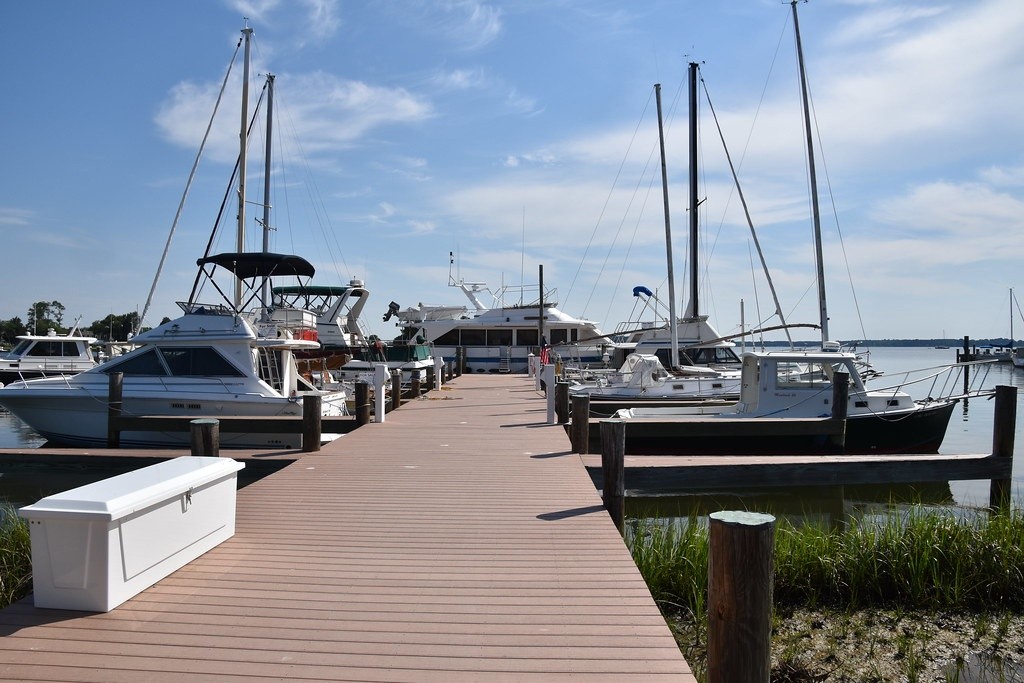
[381,250,616,374]
[0,313,101,386]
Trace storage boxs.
[17,454,246,613]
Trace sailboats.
[1,16,435,450]
[955,287,1024,366]
[568,1,997,454]
